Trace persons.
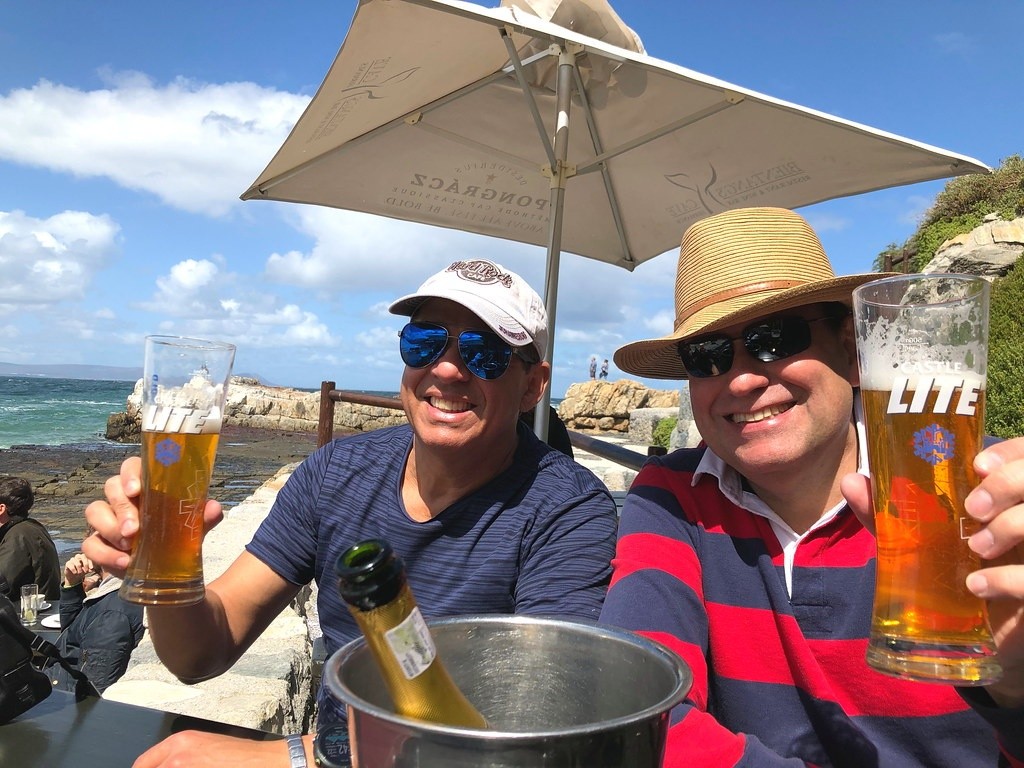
[82,256,620,768]
[41,518,146,698]
[589,358,610,380]
[584,203,1024,768]
[0,473,62,603]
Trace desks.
[14,599,67,687]
[0,682,286,768]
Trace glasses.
[397,318,539,382]
[677,311,842,378]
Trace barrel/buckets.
[326,613,693,768]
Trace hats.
[390,257,549,363]
[613,207,910,379]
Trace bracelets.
[282,734,307,768]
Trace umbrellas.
[233,1,1000,479]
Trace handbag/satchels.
[0,593,90,726]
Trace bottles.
[334,538,495,731]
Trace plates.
[41,614,61,628]
[37,603,51,610]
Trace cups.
[117,335,237,607]
[21,584,38,626]
[852,273,1004,687]
[20,594,45,608]
